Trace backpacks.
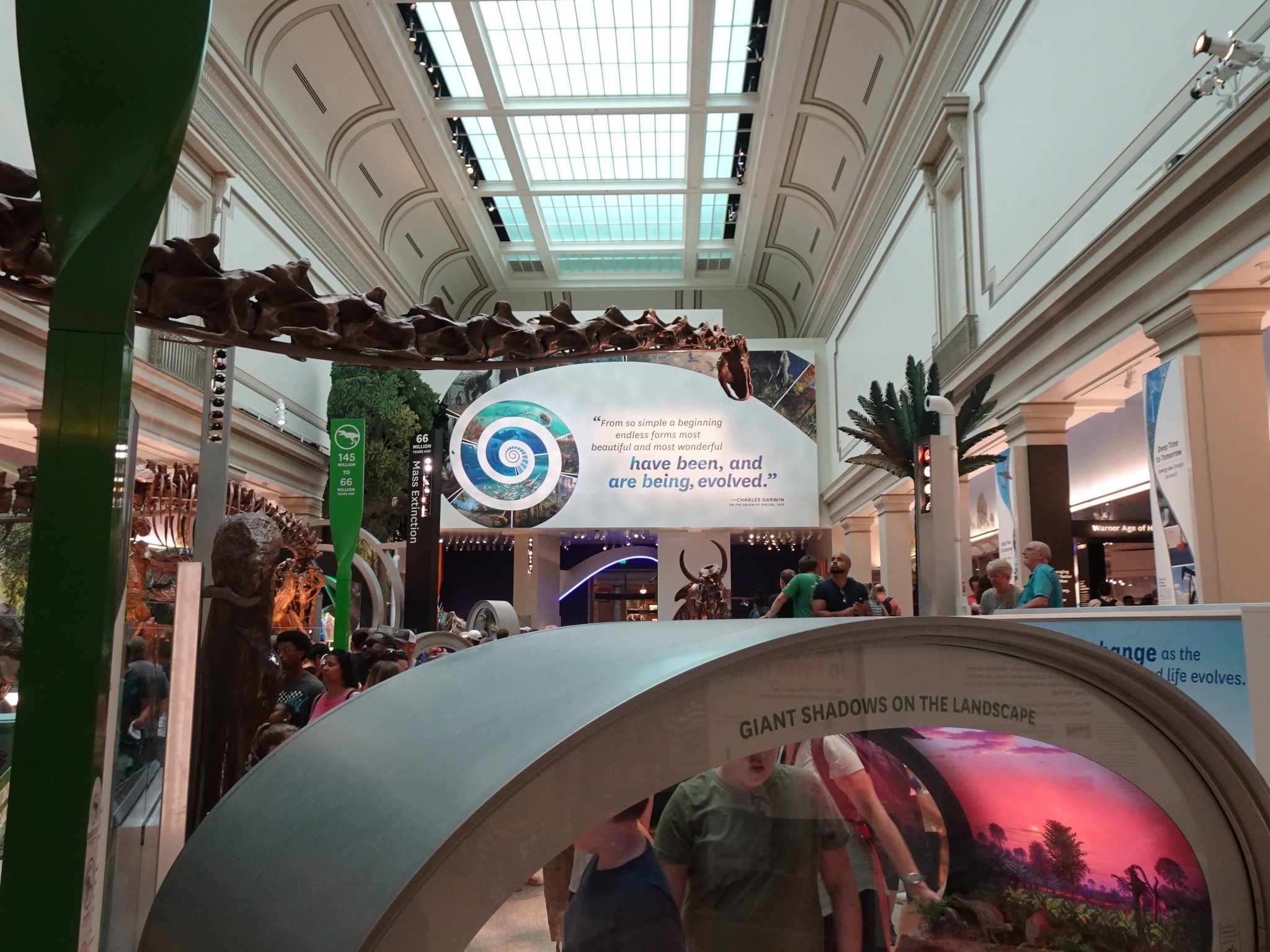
[881,597,894,616]
[812,731,926,853]
[1098,598,1118,606]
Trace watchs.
[904,871,924,886]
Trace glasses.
[385,648,405,658]
[987,576,1001,579]
[1022,548,1041,553]
[363,639,389,648]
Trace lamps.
[1103,511,1111,517]
[640,585,646,594]
[438,534,475,551]
[1123,367,1138,390]
[574,532,587,539]
[1189,28,1265,107]
[422,472,431,517]
[209,349,226,443]
[562,544,568,550]
[492,535,514,551]
[594,531,608,540]
[476,535,490,551]
[603,544,609,550]
[739,530,825,552]
[623,530,645,546]
[275,399,287,428]
[403,3,496,213]
[727,14,767,220]
[612,542,615,548]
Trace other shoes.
[527,873,543,885]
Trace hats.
[393,629,416,645]
[462,630,482,641]
[545,625,557,629]
[519,626,531,633]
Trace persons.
[513,734,942,952]
[747,540,1160,618]
[116,624,560,779]
